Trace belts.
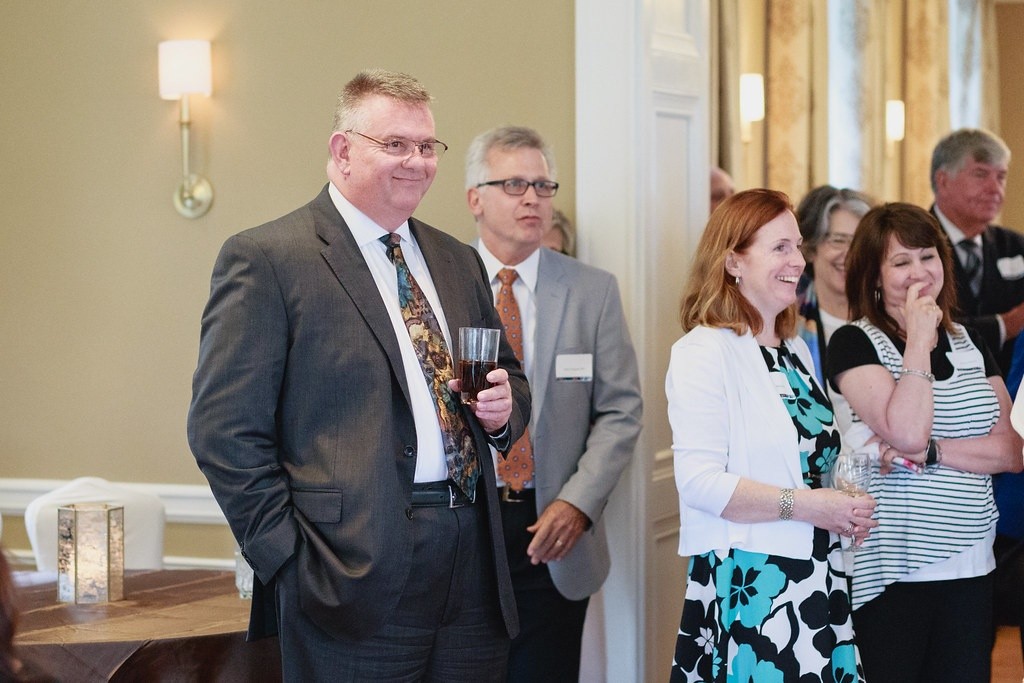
[411,482,476,508]
[497,486,537,502]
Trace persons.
[824,201,1024,683]
[792,186,873,391]
[187,68,533,683]
[926,127,1024,623]
[467,127,643,683]
[666,187,879,683]
[711,167,736,214]
[539,208,575,255]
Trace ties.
[379,233,478,503]
[496,268,534,492]
[959,240,984,299]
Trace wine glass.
[828,453,873,552]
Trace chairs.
[24,476,166,571]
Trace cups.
[457,326,501,405]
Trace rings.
[883,439,893,449]
[933,305,938,310]
[557,541,566,547]
[844,523,856,533]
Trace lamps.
[739,71,765,142]
[885,101,904,142]
[158,37,212,217]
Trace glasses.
[345,130,448,161]
[477,178,559,197]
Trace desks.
[12,567,283,682]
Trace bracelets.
[778,488,794,521]
[899,369,935,383]
[918,439,943,473]
